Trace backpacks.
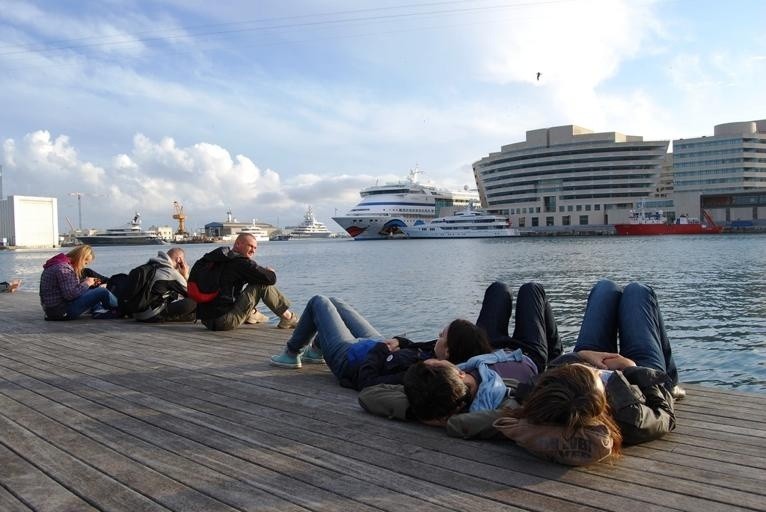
[118,260,170,313]
[187,246,242,303]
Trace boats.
[75,211,163,245]
[398,208,521,238]
[269,229,292,241]
[220,218,271,243]
[291,206,334,238]
[182,235,215,243]
[614,206,725,236]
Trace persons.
[270,280,686,457]
[0,279,22,293]
[39,233,300,331]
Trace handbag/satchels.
[493,417,613,466]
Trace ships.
[329,161,482,241]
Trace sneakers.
[671,385,686,399]
[92,309,112,318]
[277,312,299,328]
[246,308,269,323]
[270,345,326,369]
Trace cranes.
[69,191,110,229]
